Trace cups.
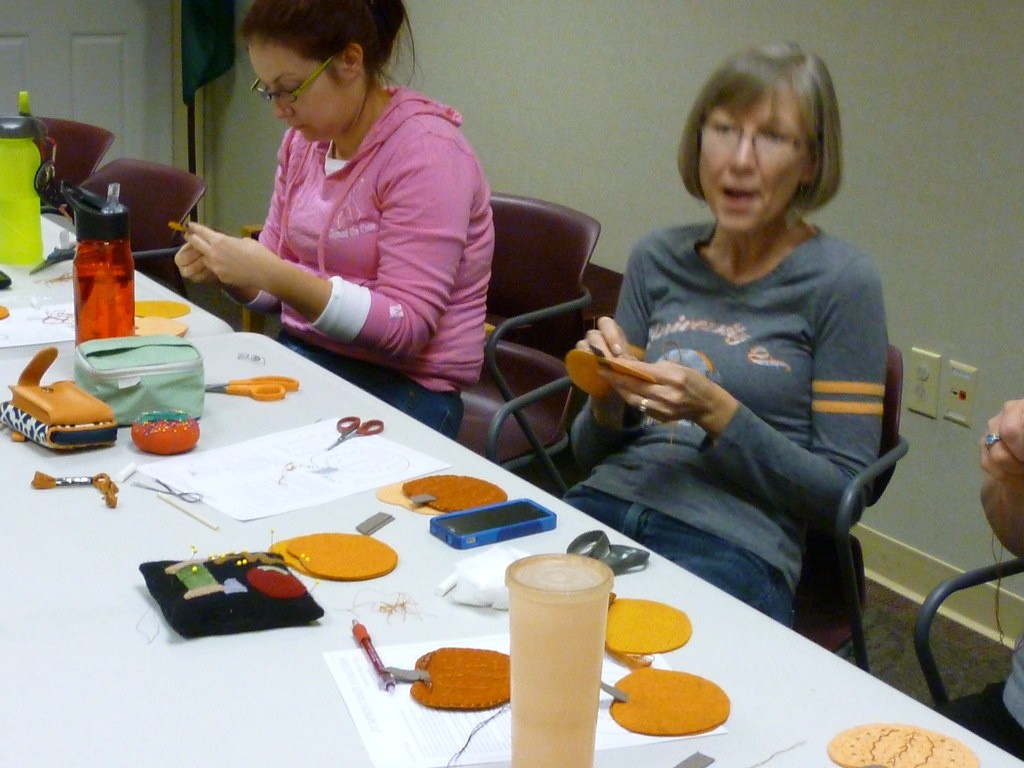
[505,553,615,768]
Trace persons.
[174,0,495,440]
[563,40,887,630]
[953,398,1024,761]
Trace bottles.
[30,160,135,359]
[0,87,46,267]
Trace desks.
[0,332,1024,768]
[0,215,234,362]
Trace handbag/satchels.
[73,333,205,426]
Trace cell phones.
[417,498,558,549]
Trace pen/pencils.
[351,619,396,692]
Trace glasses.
[250,57,333,104]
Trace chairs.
[454,193,603,498]
[484,342,908,672]
[35,115,115,221]
[80,156,206,300]
[913,556,1024,708]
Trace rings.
[985,433,1001,450]
[640,398,650,412]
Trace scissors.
[29,242,76,275]
[205,376,300,400]
[327,416,384,450]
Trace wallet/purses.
[2,346,117,451]
